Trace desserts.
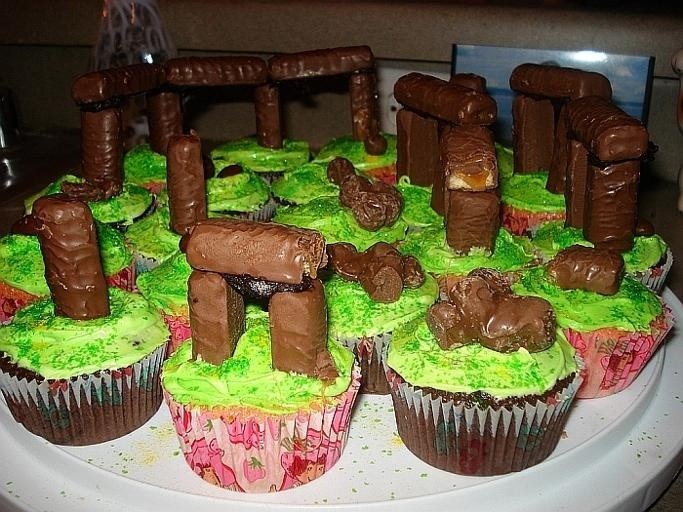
[0,130,676,494]
[10,47,658,420]
